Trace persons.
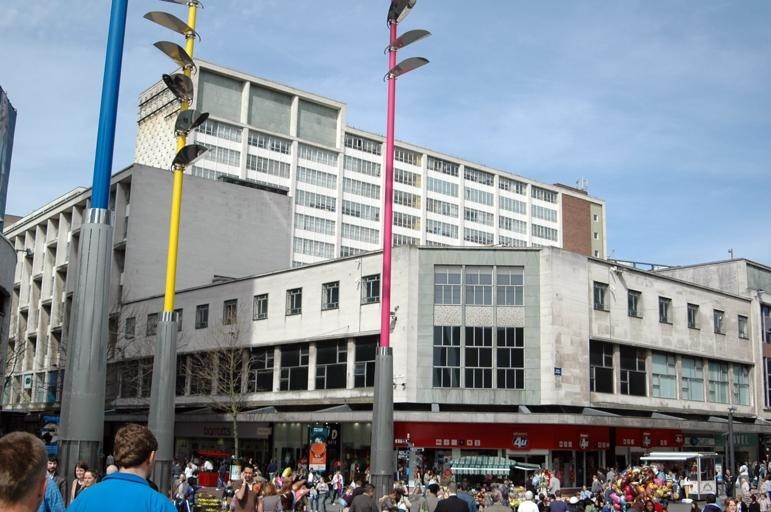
[2,422,771,512]
